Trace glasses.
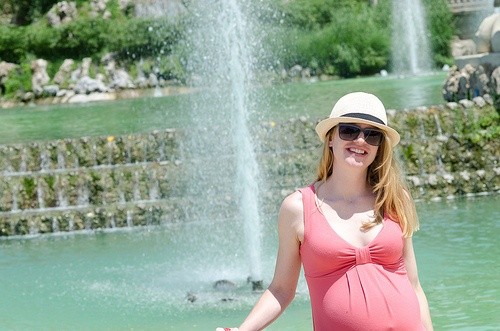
[339,123,385,146]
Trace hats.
[315,92,400,148]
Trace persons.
[214,92,440,331]
